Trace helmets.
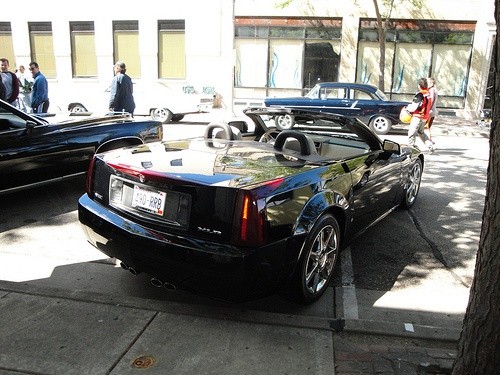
[399,106,412,123]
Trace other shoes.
[429,146,436,155]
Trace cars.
[56,79,214,124]
[0,99,163,196]
[78,107,425,304]
[264,82,412,135]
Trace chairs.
[214,126,242,140]
[283,133,319,155]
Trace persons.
[406,78,438,156]
[108,60,136,117]
[425,77,438,150]
[0,57,19,109]
[15,65,35,114]
[28,60,50,113]
